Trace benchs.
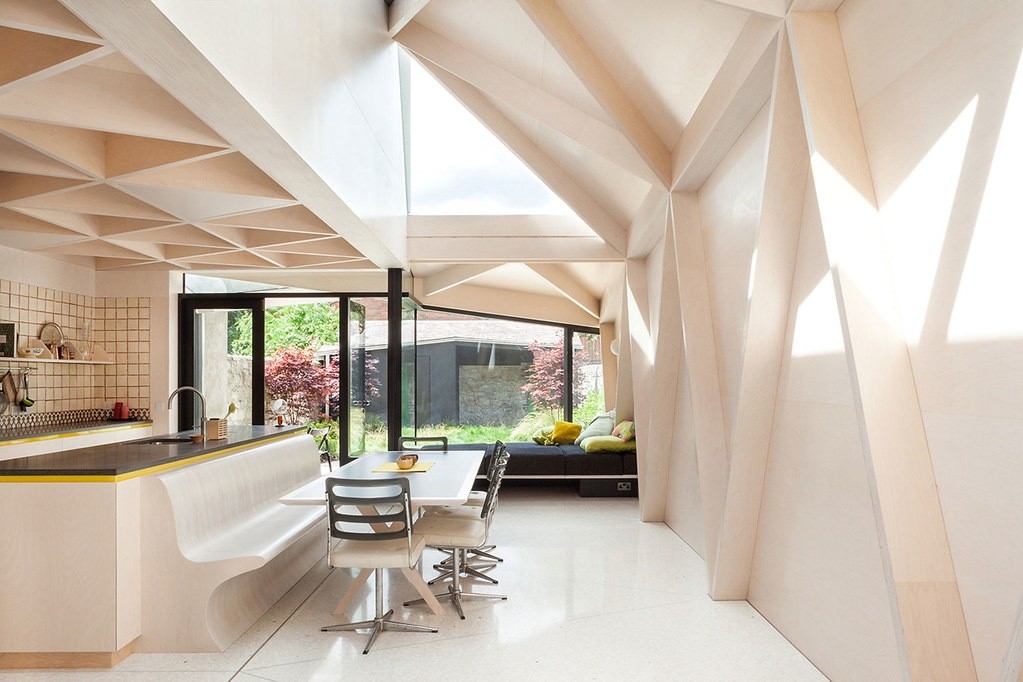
[139,435,343,652]
[421,441,638,498]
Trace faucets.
[168,386,210,442]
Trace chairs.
[398,436,448,452]
[321,477,438,655]
[307,425,332,472]
[404,439,510,619]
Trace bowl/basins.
[17,347,45,358]
[399,454,418,466]
[189,435,205,443]
[396,458,413,469]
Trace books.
[0,322,17,357]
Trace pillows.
[531,410,636,453]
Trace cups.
[114,402,124,419]
[121,406,129,419]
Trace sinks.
[120,438,193,447]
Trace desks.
[278,449,485,615]
